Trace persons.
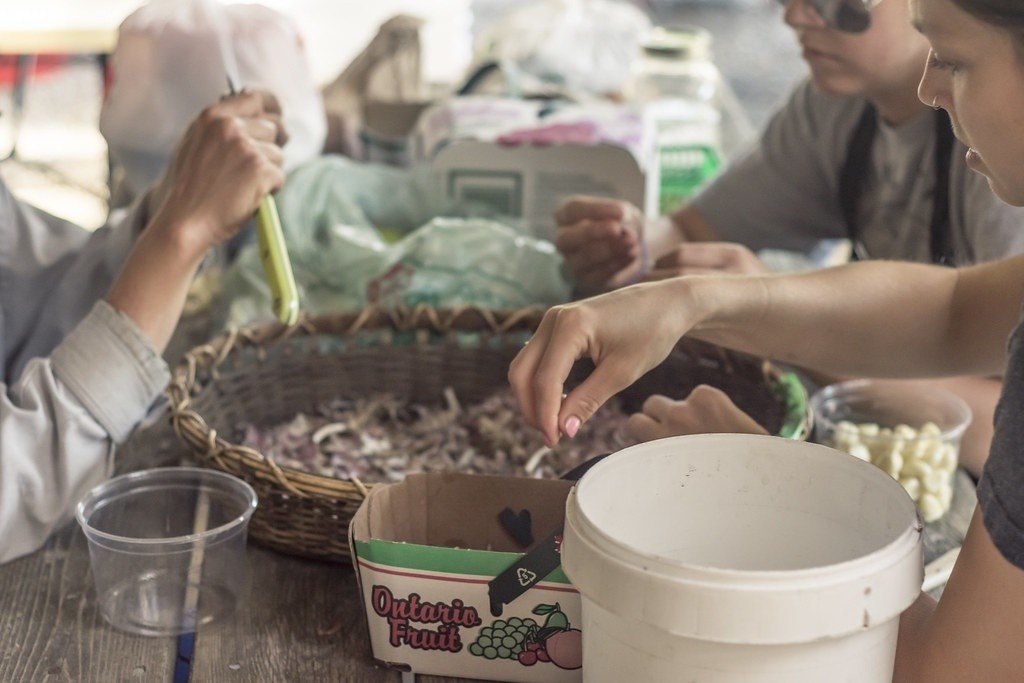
[506,1,1024,682]
[0,90,288,562]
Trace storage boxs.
[354,24,727,245]
[347,471,584,682]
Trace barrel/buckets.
[559,432,924,683]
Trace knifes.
[226,79,299,327]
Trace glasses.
[776,0,882,33]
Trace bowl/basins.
[814,380,972,522]
[75,468,257,636]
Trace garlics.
[824,419,957,523]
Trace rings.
[523,341,528,345]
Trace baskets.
[162,301,814,566]
